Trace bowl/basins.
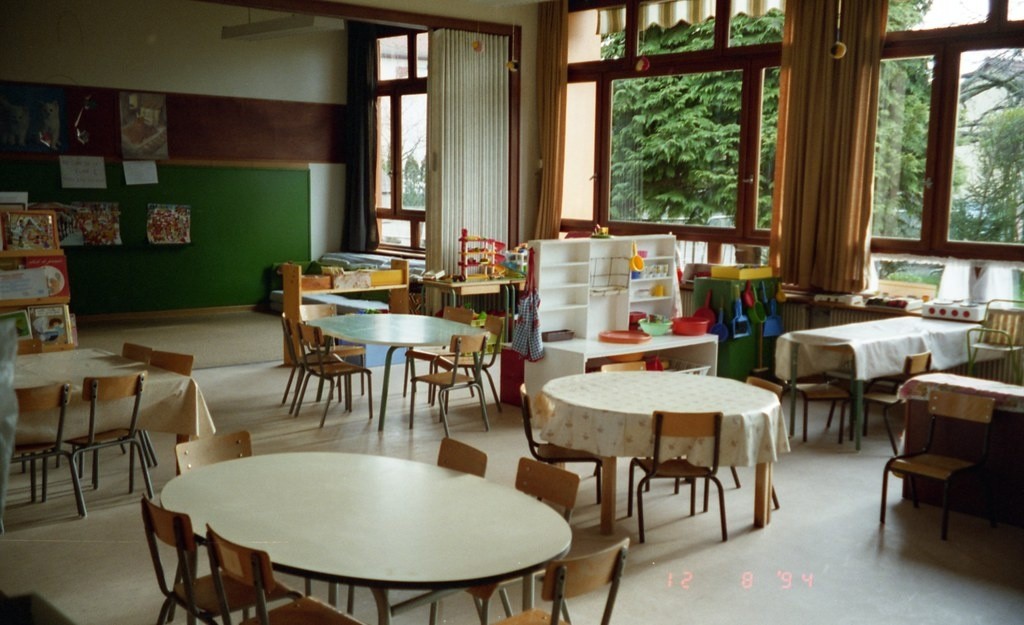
[672,317,709,335]
[608,352,644,363]
[638,318,673,335]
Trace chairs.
[0,259,1024,625]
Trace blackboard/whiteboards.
[0,160,310,323]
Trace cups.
[598,227,608,236]
[632,251,669,297]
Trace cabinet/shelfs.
[525,233,720,398]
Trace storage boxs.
[922,302,988,322]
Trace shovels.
[732,300,751,338]
[763,299,782,337]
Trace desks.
[535,373,790,536]
[898,370,1024,528]
[161,453,569,625]
[279,257,411,369]
[11,349,216,449]
[303,314,502,432]
[774,315,1005,449]
[416,277,526,321]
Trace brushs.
[736,301,747,333]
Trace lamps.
[221,9,347,37]
